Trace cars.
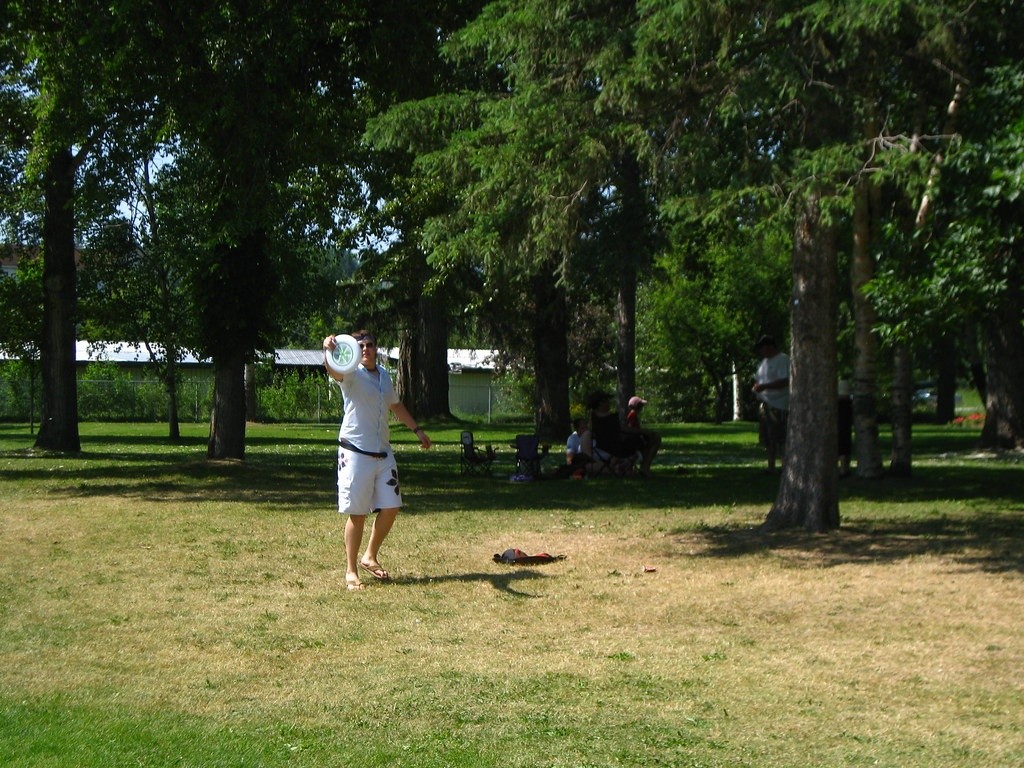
[886,379,964,409]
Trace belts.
[336,438,388,458]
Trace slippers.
[346,572,366,591]
[358,561,388,579]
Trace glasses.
[359,342,373,349]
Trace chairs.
[460,431,498,477]
[510,435,545,480]
[590,430,622,479]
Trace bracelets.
[414,426,421,433]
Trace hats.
[587,390,614,408]
[628,396,648,407]
[755,334,776,349]
[501,548,528,560]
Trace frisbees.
[324,334,362,376]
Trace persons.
[585,390,615,433]
[839,385,856,476]
[623,392,661,477]
[559,418,597,472]
[323,330,432,589]
[752,335,790,472]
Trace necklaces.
[367,368,377,373]
